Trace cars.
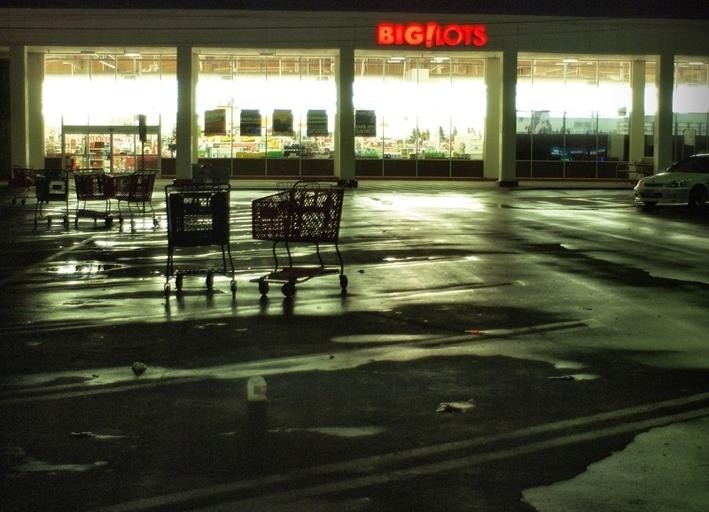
[628,152,709,210]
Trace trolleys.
[243,175,359,296]
[160,177,239,298]
[7,159,160,227]
